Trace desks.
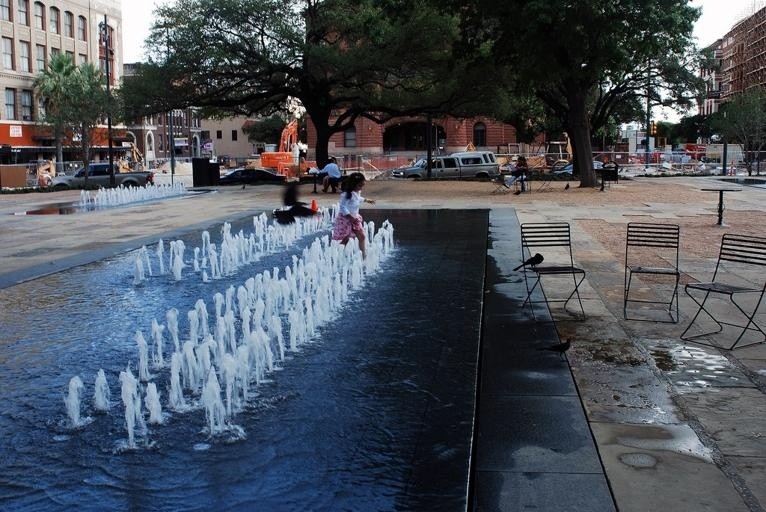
[701,189,742,228]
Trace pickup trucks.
[46,162,154,192]
[391,156,499,181]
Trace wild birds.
[241,185,245,190]
[562,183,569,192]
[511,253,544,271]
[273,181,318,225]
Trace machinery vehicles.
[261,118,300,171]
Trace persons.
[316,160,341,193]
[331,172,378,261]
[281,166,301,208]
[331,156,337,165]
[515,156,530,192]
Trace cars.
[553,160,604,177]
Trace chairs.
[521,223,766,351]
[488,167,557,195]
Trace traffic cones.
[311,199,318,214]
